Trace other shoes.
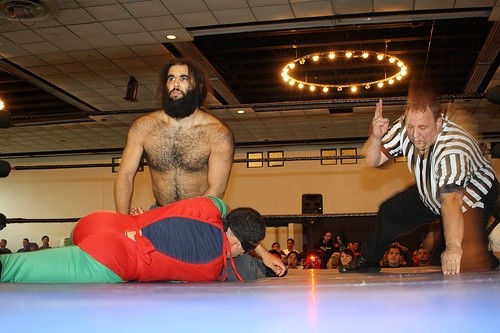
[262,262,288,277]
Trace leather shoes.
[338,252,381,273]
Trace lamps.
[279,50,410,93]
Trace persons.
[267,230,369,269]
[16,236,51,253]
[0,195,287,283]
[113,58,235,215]
[64,238,72,246]
[0,239,12,254]
[338,90,500,275]
[379,238,433,268]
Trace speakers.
[302,194,323,227]
